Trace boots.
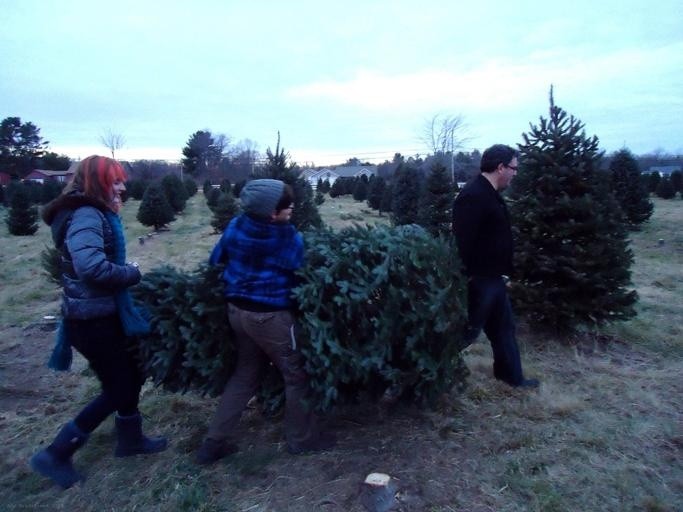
[29,420,90,489]
[114,410,168,458]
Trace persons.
[195,179,338,465]
[29,155,167,487]
[446,144,539,389]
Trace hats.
[239,178,284,218]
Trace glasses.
[504,163,518,172]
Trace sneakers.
[196,442,240,464]
[518,378,540,389]
